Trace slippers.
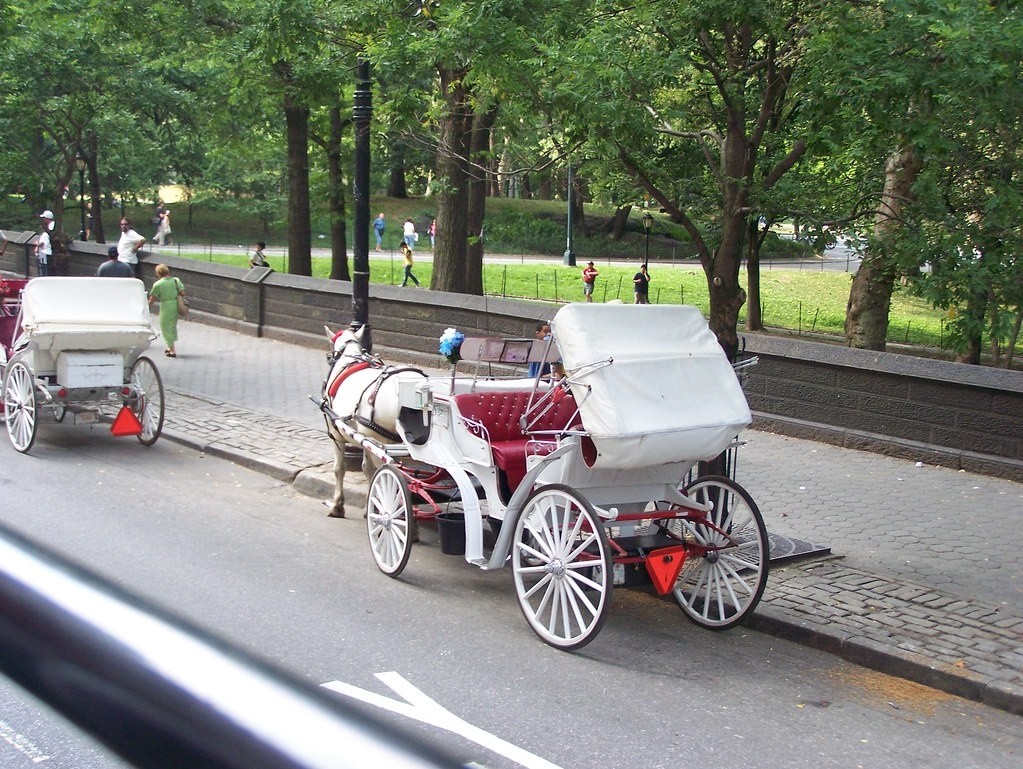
[165,349,176,357]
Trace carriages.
[303,299,772,651]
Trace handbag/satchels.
[175,278,189,315]
[263,262,269,267]
[379,229,384,235]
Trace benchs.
[451,394,587,500]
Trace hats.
[40,210,54,219]
[587,261,594,265]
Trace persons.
[371,212,386,253]
[0,230,7,256]
[117,216,146,276]
[582,261,599,302]
[148,264,186,357]
[403,217,416,255]
[427,218,435,248]
[249,240,267,269]
[86,202,92,241]
[151,199,174,245]
[632,264,650,304]
[398,240,420,288]
[33,210,55,276]
[526,322,563,382]
[96,247,135,278]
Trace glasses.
[121,223,130,227]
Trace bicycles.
[798,234,838,250]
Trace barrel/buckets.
[485,513,530,550]
[435,489,465,555]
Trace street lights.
[642,211,655,270]
[563,128,577,266]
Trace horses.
[324,323,429,518]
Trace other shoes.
[398,284,406,288]
[416,281,420,288]
